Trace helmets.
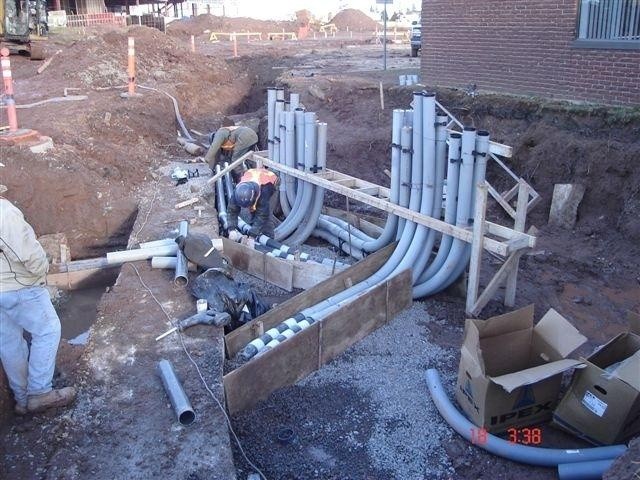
[184,233,222,268]
[234,182,256,207]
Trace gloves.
[229,230,254,248]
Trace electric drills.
[155,309,231,341]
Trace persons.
[205,125,259,183]
[0,184,77,416]
[226,167,280,248]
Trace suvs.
[410,22,421,56]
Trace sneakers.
[15,386,76,415]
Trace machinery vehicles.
[0,1,49,60]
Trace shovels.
[194,206,205,217]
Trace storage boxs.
[551,330,640,447]
[454,303,589,438]
